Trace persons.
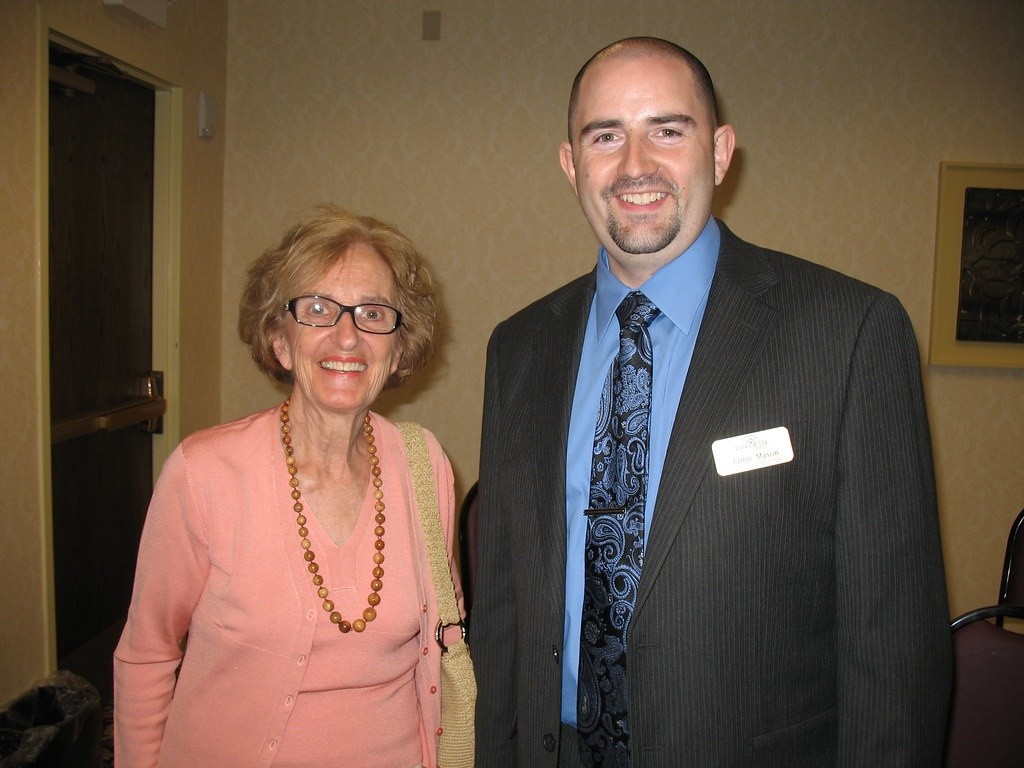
[112,204,467,768]
[469,36,951,768]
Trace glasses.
[280,296,403,334]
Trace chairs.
[941,515,1024,768]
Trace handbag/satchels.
[436,640,476,768]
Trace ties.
[577,293,661,767]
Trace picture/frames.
[928,162,1024,370]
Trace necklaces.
[281,396,386,633]
